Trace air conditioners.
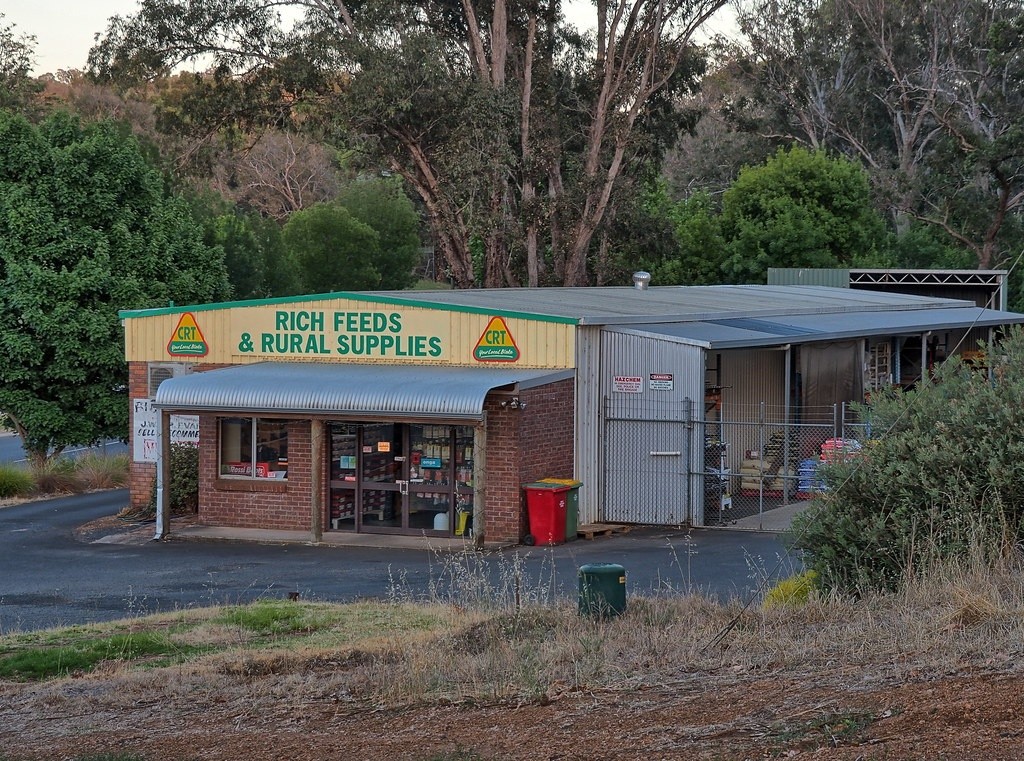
[148,362,193,400]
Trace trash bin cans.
[521,478,584,546]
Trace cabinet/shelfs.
[332,429,381,462]
